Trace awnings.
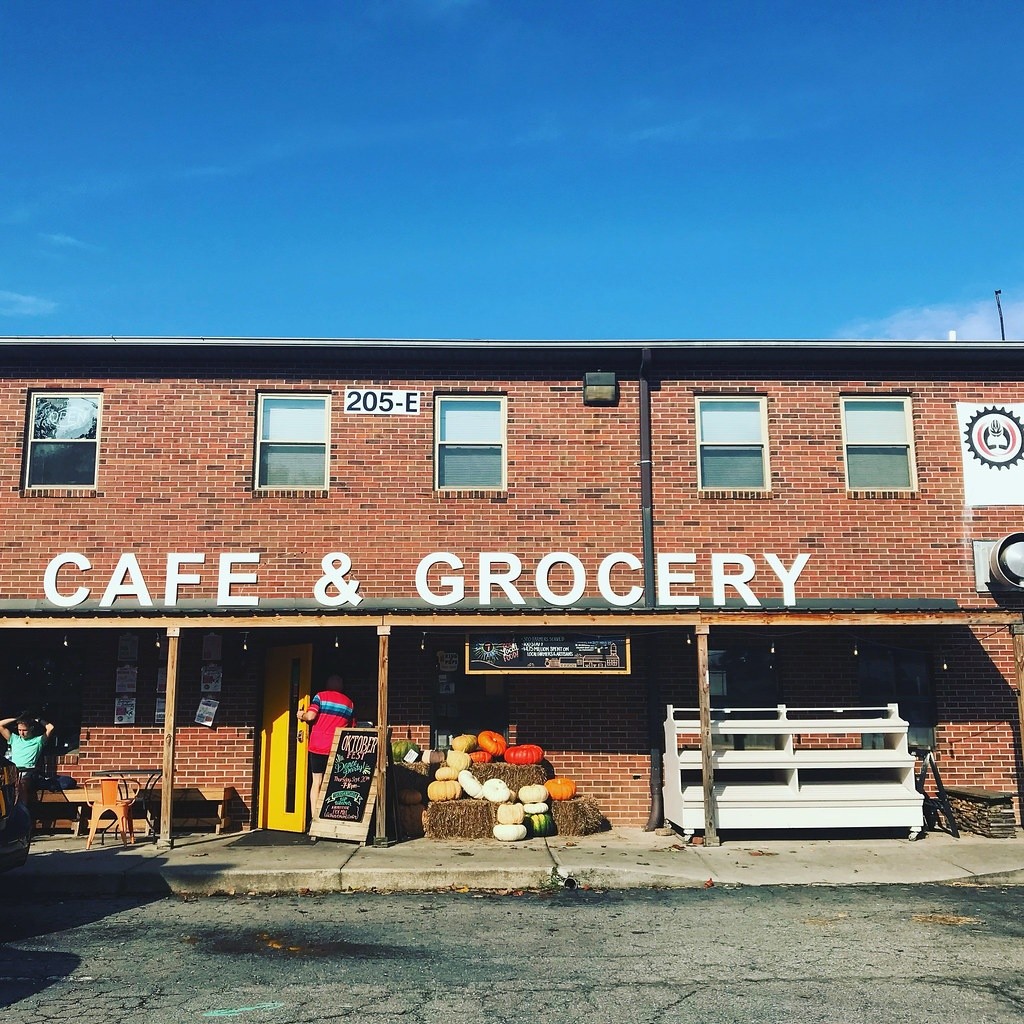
[0,598,1024,849]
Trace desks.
[93,768,163,845]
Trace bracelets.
[38,718,42,723]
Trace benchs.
[32,786,235,836]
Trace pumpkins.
[421,730,577,841]
[398,787,422,805]
[390,740,420,762]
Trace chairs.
[83,777,141,850]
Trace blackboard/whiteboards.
[464,632,632,676]
[318,727,381,824]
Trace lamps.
[583,372,617,406]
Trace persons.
[296,674,356,836]
[0,710,54,806]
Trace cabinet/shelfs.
[662,703,925,843]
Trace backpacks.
[32,772,77,791]
